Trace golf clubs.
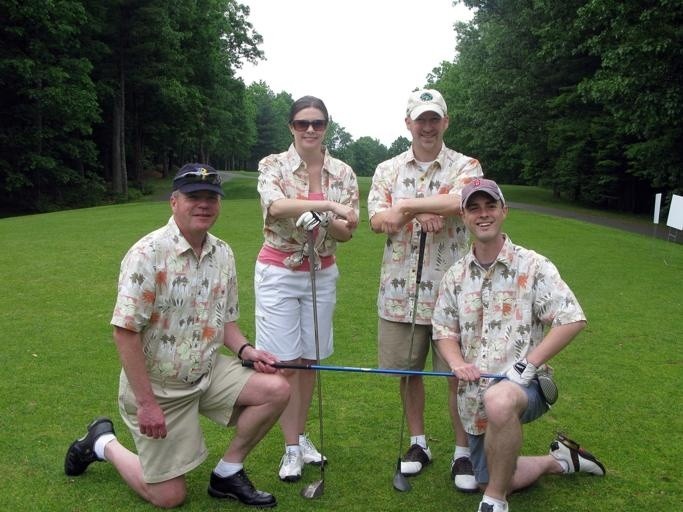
[242,360,558,405]
[299,231,324,499]
[393,228,427,492]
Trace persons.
[368,89,485,493]
[253,95,360,482]
[65,162,292,509]
[430,178,606,512]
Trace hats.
[172,162,225,198]
[406,88,448,122]
[459,178,506,212]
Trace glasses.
[289,119,329,132]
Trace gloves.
[504,358,536,386]
[298,210,330,231]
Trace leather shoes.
[206,467,277,508]
[400,444,433,476]
[64,416,117,476]
[450,456,480,492]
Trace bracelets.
[238,343,255,360]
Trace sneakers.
[298,432,328,466]
[477,499,510,512]
[279,444,305,481]
[549,432,606,477]
